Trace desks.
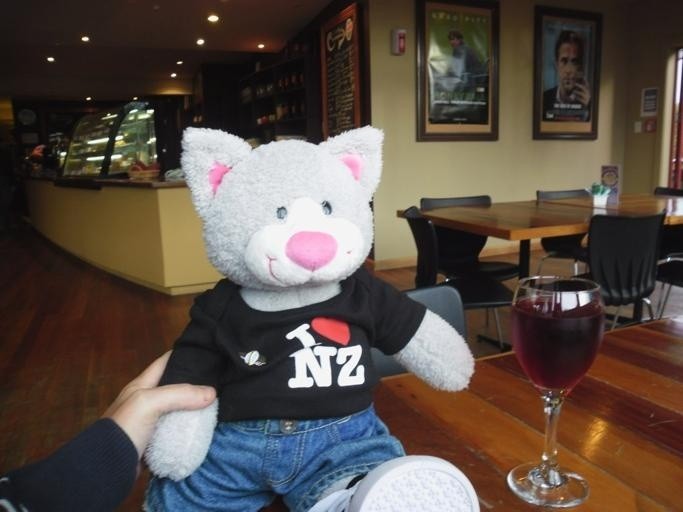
[397,192,683,280]
[372,318,683,512]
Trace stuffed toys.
[141,125,481,512]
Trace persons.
[542,30,592,121]
[0,348,217,512]
[448,29,487,77]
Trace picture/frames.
[532,5,602,140]
[314,0,370,146]
[415,0,500,142]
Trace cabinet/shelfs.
[61,95,156,179]
[236,81,306,128]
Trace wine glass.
[506,276,603,508]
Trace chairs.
[654,187,683,196]
[421,195,519,329]
[658,248,683,321]
[654,187,683,267]
[574,208,667,331]
[370,285,464,379]
[402,206,513,353]
[537,188,592,277]
